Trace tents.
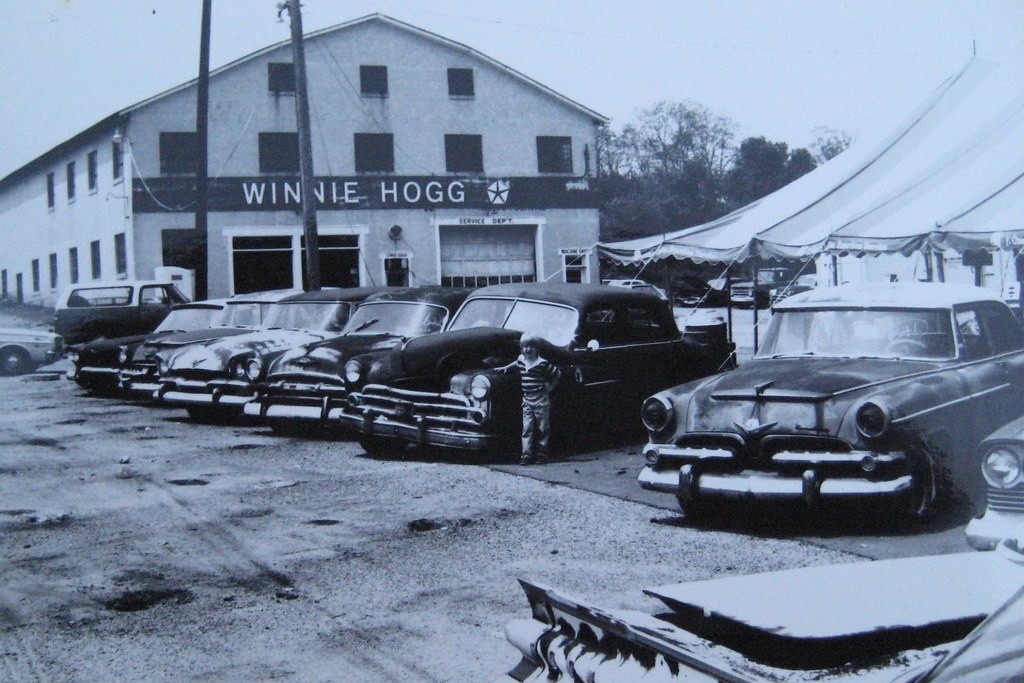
[596,40,1023,279]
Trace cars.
[731,266,796,312]
[962,416,1024,559]
[51,280,234,402]
[605,279,674,312]
[0,326,63,378]
[673,278,729,310]
[340,283,736,463]
[638,281,1024,525]
[152,282,387,424]
[769,275,819,313]
[499,541,1024,682]
[243,285,478,440]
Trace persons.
[494,331,561,466]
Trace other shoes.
[535,450,549,464]
[520,451,535,465]
